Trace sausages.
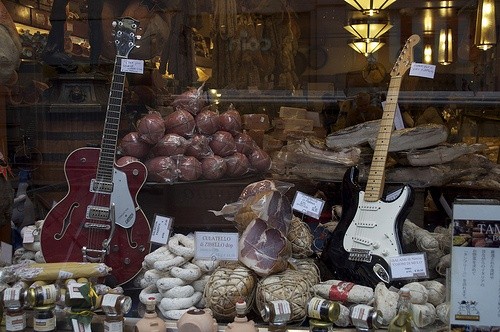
[137,234,225,322]
[0,261,124,310]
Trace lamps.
[437,26,454,66]
[344,0,397,60]
[475,0,497,51]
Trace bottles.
[134,297,167,332]
[386,289,420,332]
[225,300,259,332]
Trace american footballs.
[121,89,272,182]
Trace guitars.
[39,15,152,286]
[319,33,421,288]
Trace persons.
[322,94,340,135]
[346,94,383,127]
[417,103,443,124]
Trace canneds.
[305,296,384,332]
[0,281,131,332]
